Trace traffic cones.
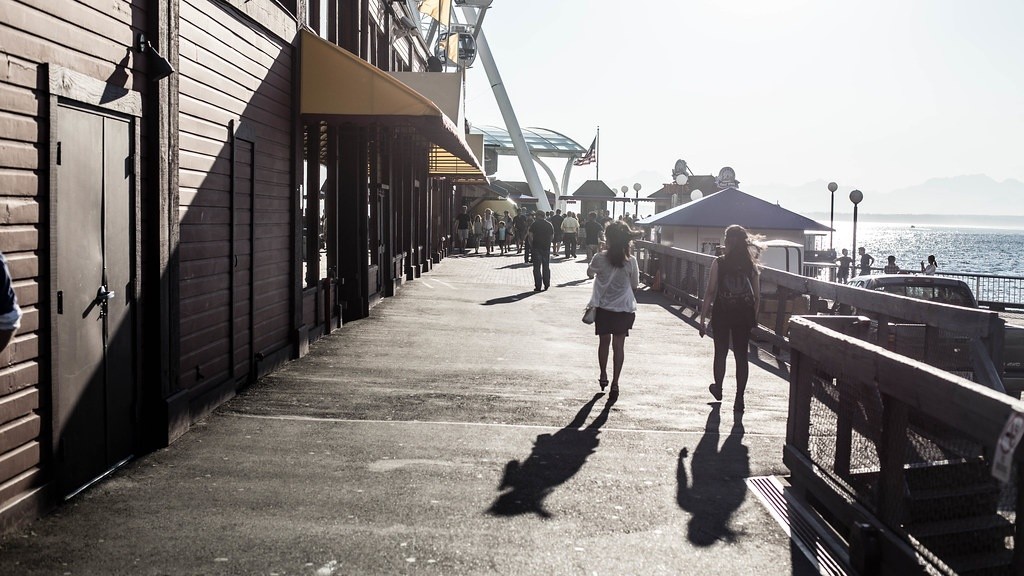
[650,267,663,292]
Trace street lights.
[611,187,619,220]
[676,173,688,204]
[848,188,864,279]
[620,185,629,218]
[633,182,642,218]
[827,182,838,249]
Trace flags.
[574,136,596,166]
[440,33,457,62]
[419,0,450,27]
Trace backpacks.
[712,258,758,330]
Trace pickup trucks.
[817,271,1024,401]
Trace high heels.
[609,384,619,400]
[599,379,609,391]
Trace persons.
[833,249,852,284]
[857,247,875,275]
[0,254,22,353]
[884,255,900,274]
[921,255,938,276]
[586,221,640,396]
[699,226,765,409]
[454,205,651,292]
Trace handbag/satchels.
[582,304,596,324]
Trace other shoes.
[709,384,722,400]
[735,401,744,410]
[534,288,541,292]
[545,286,548,290]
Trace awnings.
[301,29,490,188]
